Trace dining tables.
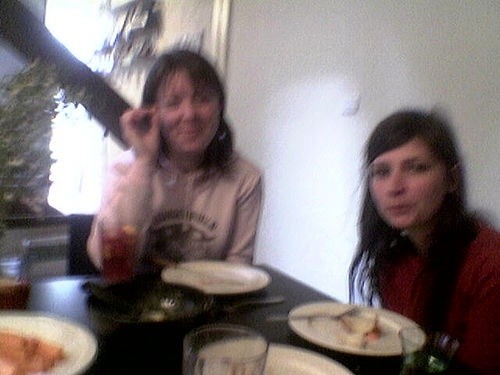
[0,265,480,375]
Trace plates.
[289,301,426,356]
[0,315,98,375]
[200,342,353,375]
[160,261,270,295]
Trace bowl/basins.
[86,279,213,333]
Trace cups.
[182,322,270,375]
[0,256,28,312]
